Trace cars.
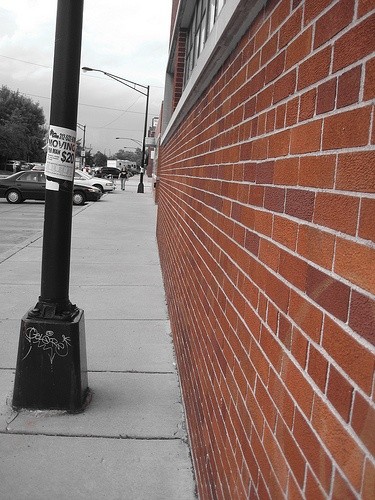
[0,171,102,205]
[6,160,45,171]
[73,169,115,193]
[94,167,129,178]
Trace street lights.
[81,67,149,193]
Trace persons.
[11,161,17,175]
[117,166,128,191]
[37,163,44,171]
